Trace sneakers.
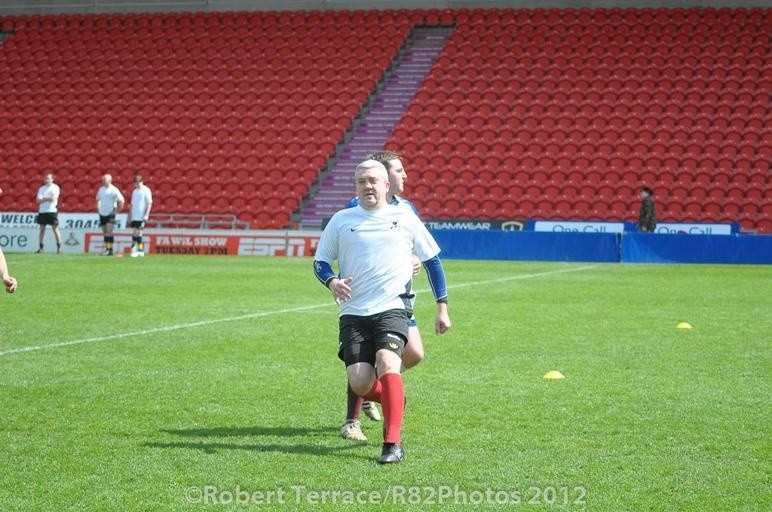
[130,250,145,258]
[362,401,381,421]
[378,442,404,464]
[340,419,368,441]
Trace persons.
[35,174,62,254]
[638,186,657,232]
[128,174,153,258]
[94,174,125,256]
[338,150,425,442]
[312,160,452,464]
[0,246,18,294]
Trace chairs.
[0,4,771,238]
